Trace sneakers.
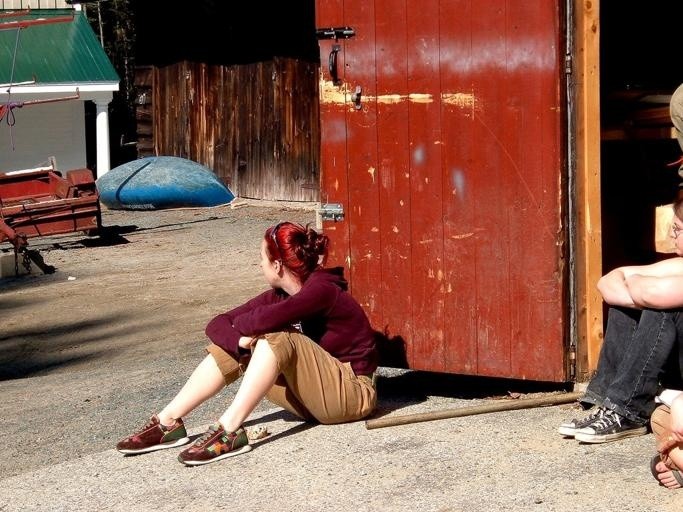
[557,407,647,444]
[177,421,253,465]
[117,414,190,456]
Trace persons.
[648,390,683,490]
[558,185,683,443]
[116,221,378,467]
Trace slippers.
[650,453,682,489]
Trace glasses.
[671,225,682,239]
